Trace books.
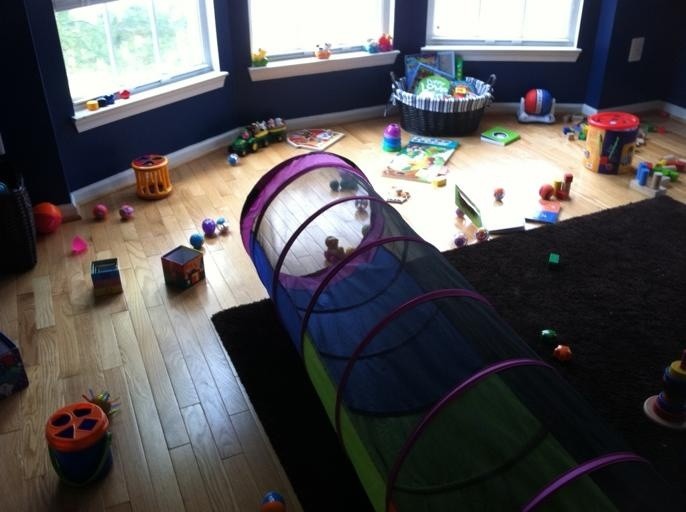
[405,52,476,99]
[385,142,457,184]
[480,126,520,147]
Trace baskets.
[389,71,497,137]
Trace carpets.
[210,194,686,510]
